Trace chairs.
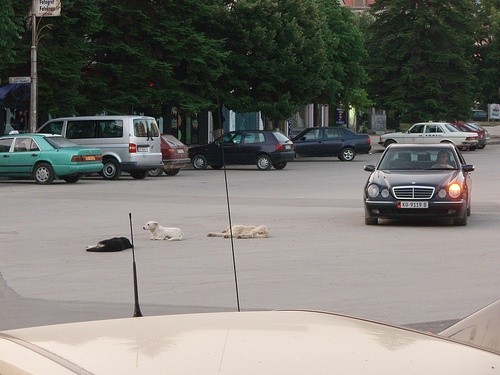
[93,124,105,138]
[430,127,439,132]
[7,144,19,152]
[103,126,112,137]
[18,143,27,151]
[254,137,260,143]
[389,158,410,169]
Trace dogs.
[143,220,184,241]
[86,236,132,252]
[207,224,269,239]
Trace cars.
[361,144,476,227]
[161,133,189,176]
[468,110,488,121]
[449,122,486,151]
[290,125,372,161]
[378,120,479,149]
[0,130,104,185]
[187,129,296,170]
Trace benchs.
[409,160,456,168]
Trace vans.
[35,115,165,180]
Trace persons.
[434,151,454,169]
